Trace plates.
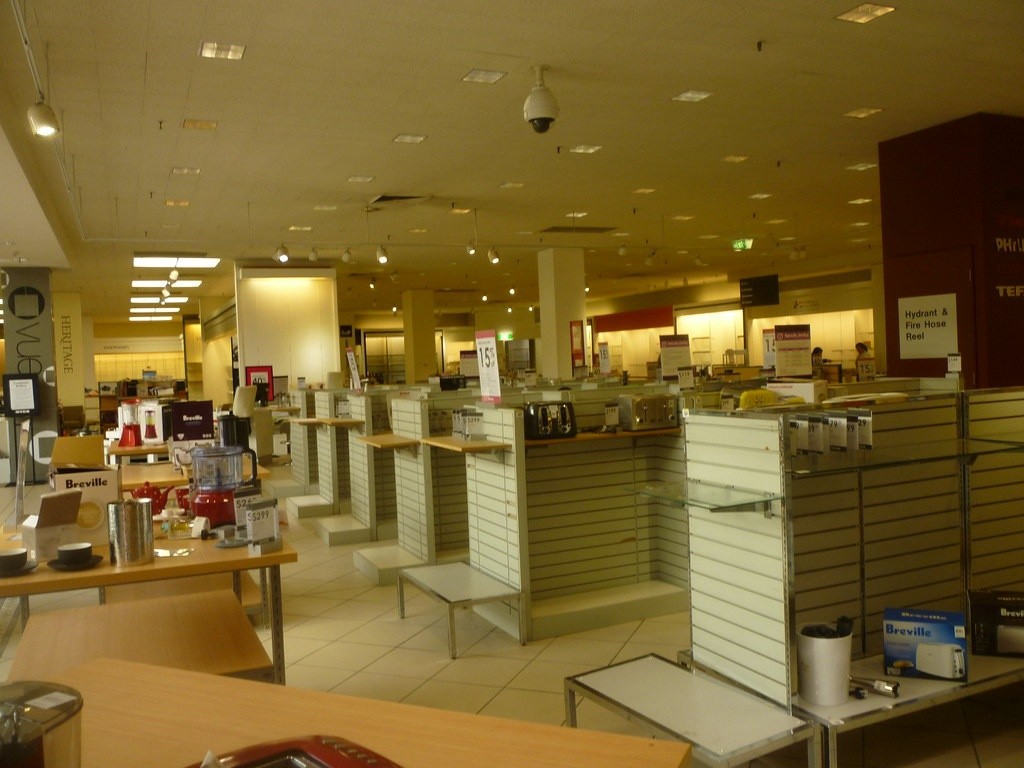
[0,560,39,578]
[214,537,251,549]
[47,555,104,571]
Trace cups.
[57,543,92,564]
[175,486,191,516]
[0,548,27,571]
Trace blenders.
[0,679,83,768]
[189,443,259,528]
[144,410,158,438]
[118,399,143,447]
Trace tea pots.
[131,481,174,516]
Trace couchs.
[61,406,86,429]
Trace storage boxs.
[42,437,121,549]
[881,607,968,682]
[762,379,827,403]
[138,402,171,445]
[20,492,84,562]
[169,400,218,466]
[966,589,1024,660]
[828,386,849,398]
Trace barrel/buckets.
[107,498,155,566]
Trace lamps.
[23,89,60,141]
[488,246,499,264]
[341,247,352,264]
[465,242,477,255]
[272,242,289,264]
[309,248,318,263]
[376,244,389,264]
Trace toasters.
[913,642,966,679]
[719,385,779,410]
[618,392,678,431]
[522,401,578,439]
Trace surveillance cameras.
[523,88,558,133]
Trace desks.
[558,652,824,768]
[394,562,527,663]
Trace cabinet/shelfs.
[0,364,1024,768]
